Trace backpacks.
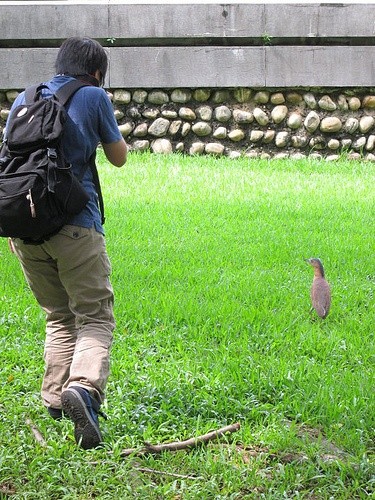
[0,77,97,244]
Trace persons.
[4,36,127,449]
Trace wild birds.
[304,257,331,319]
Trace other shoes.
[60,387,108,447]
[48,407,63,421]
